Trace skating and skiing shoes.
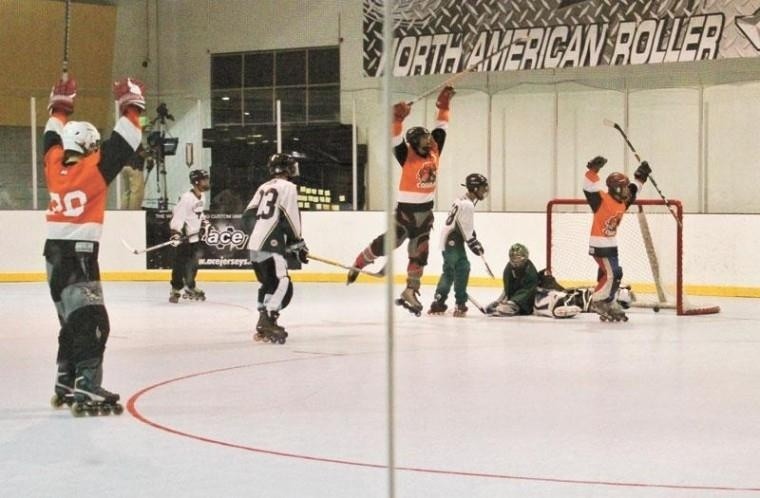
[254,313,287,343]
[347,252,369,286]
[170,288,180,303]
[396,285,468,318]
[182,287,207,301]
[591,301,629,321]
[51,361,123,417]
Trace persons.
[347,85,457,317]
[40,77,147,418]
[167,169,210,303]
[118,115,152,211]
[533,268,637,319]
[581,156,653,322]
[239,151,309,345]
[426,173,490,317]
[485,243,539,317]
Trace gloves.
[112,79,146,113]
[634,161,651,183]
[436,86,457,110]
[468,238,483,255]
[393,101,410,122]
[288,239,309,263]
[170,234,181,248]
[200,221,211,242]
[48,80,77,114]
[586,156,608,173]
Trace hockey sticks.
[407,37,527,107]
[308,256,386,277]
[602,119,686,232]
[122,231,205,255]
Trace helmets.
[61,120,101,153]
[510,243,528,268]
[607,173,629,191]
[466,175,488,192]
[189,170,209,182]
[268,153,295,178]
[407,127,430,142]
